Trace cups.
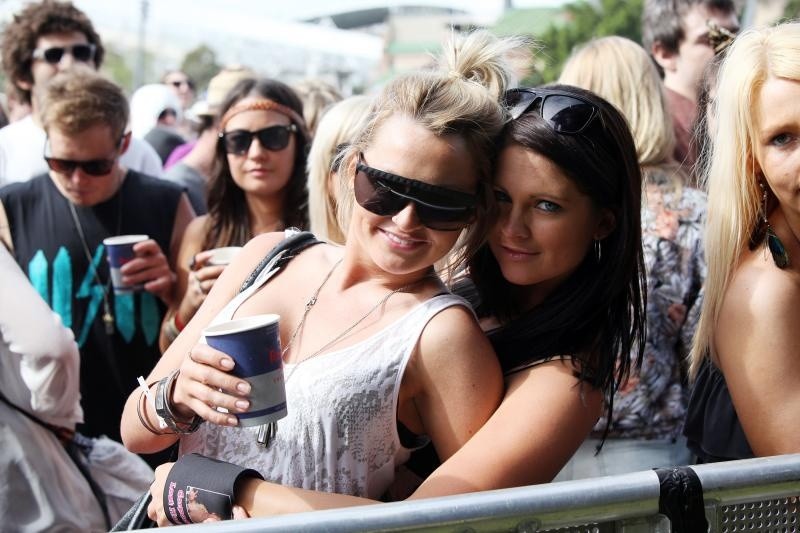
[204,314,287,428]
[200,245,242,266]
[103,233,149,295]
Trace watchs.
[155,376,201,436]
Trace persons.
[0,0,800,533]
[119,20,540,525]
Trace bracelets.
[163,321,177,342]
[137,380,173,435]
[174,313,186,332]
[163,369,201,425]
[169,315,181,336]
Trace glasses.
[30,44,94,63]
[218,125,296,155]
[43,128,124,177]
[501,86,608,159]
[173,81,194,88]
[353,164,477,231]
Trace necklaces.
[257,256,435,451]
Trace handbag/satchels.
[68,436,156,532]
